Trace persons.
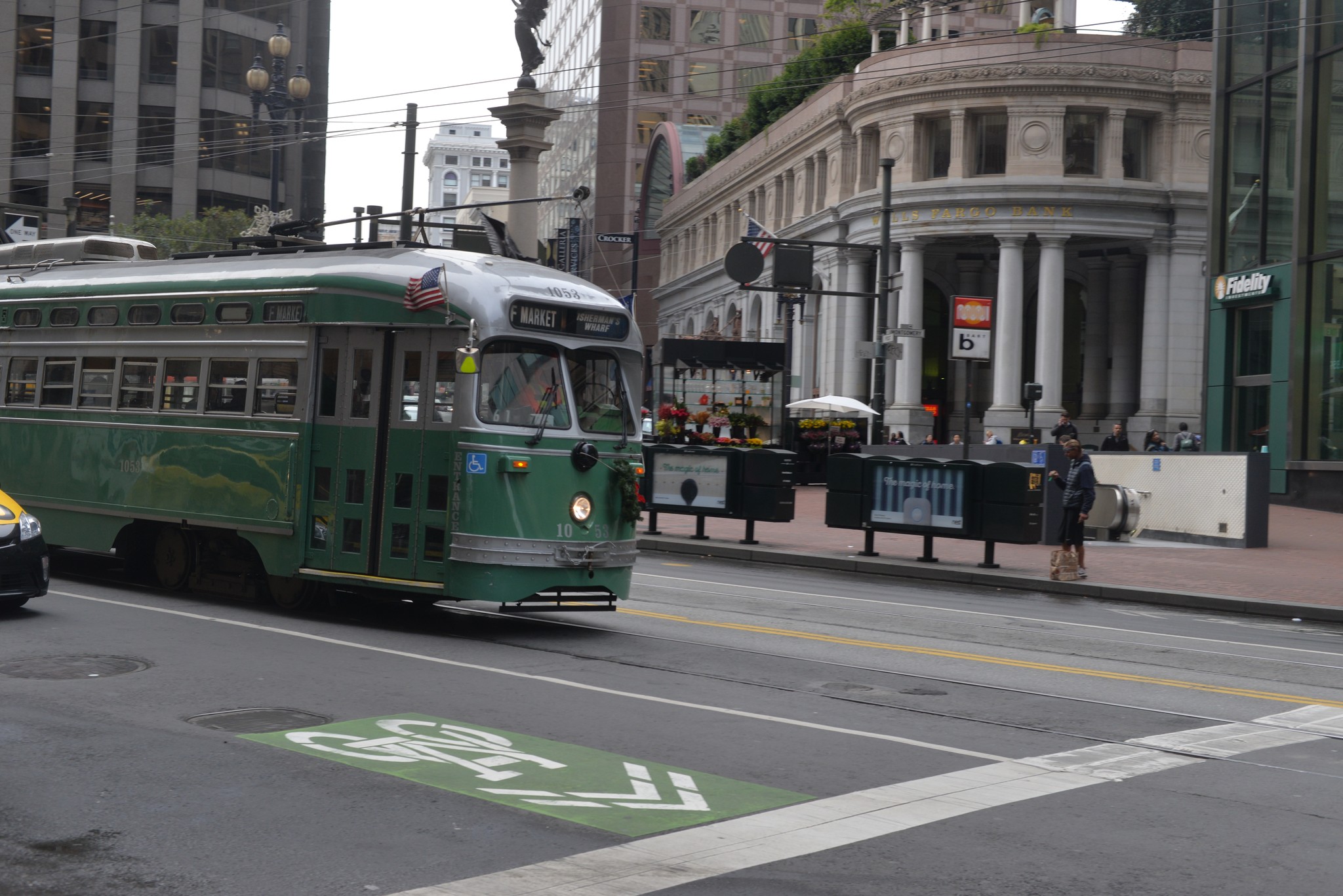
[949,434,964,445]
[223,380,247,412]
[1173,422,1199,452]
[1100,423,1129,451]
[891,431,906,445]
[1051,412,1077,444]
[185,387,199,410]
[512,0,545,75]
[983,431,998,444]
[1049,440,1095,579]
[516,357,562,414]
[921,434,935,445]
[1144,430,1171,452]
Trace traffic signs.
[1,211,40,244]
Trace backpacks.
[1179,433,1193,452]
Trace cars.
[0,490,51,609]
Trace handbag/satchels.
[1050,549,1078,581]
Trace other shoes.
[1078,566,1087,579]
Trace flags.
[745,221,775,286]
[404,267,445,312]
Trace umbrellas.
[784,393,881,455]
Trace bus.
[0,188,650,618]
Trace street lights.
[242,21,312,235]
